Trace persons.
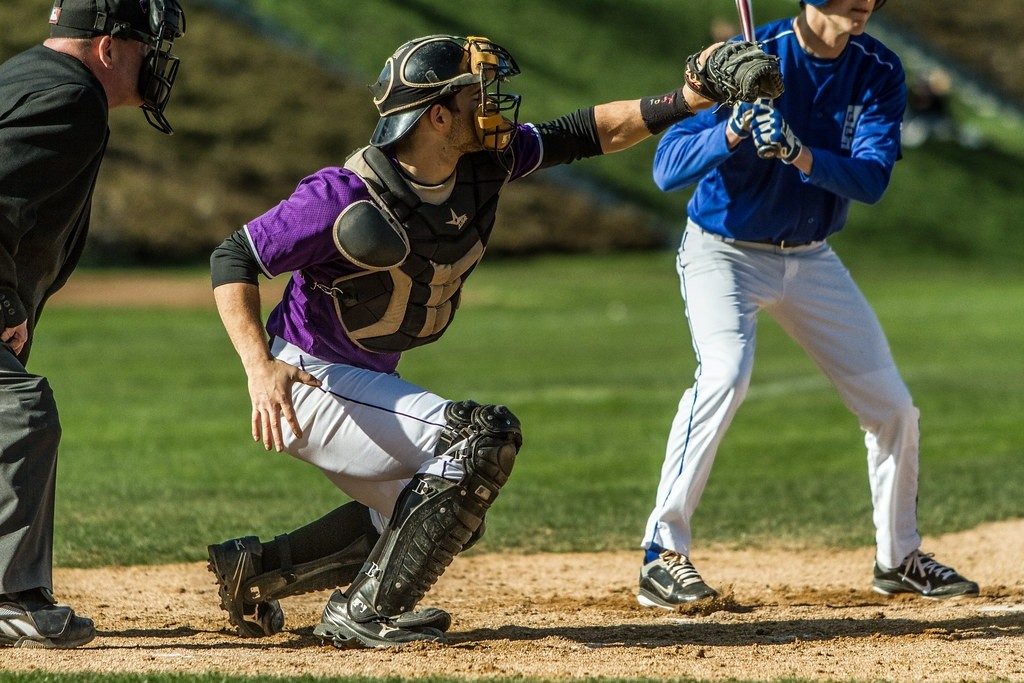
[0,0,187,649]
[637,0,980,610]
[207,34,786,649]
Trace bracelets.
[639,87,698,136]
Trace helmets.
[369,35,475,148]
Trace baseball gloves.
[682,40,786,116]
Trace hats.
[48,0,185,39]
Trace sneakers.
[870,550,979,601]
[638,552,720,611]
[207,536,281,638]
[311,588,447,651]
[0,601,96,649]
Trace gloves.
[729,100,803,165]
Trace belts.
[735,238,811,249]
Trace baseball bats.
[733,1,782,161]
